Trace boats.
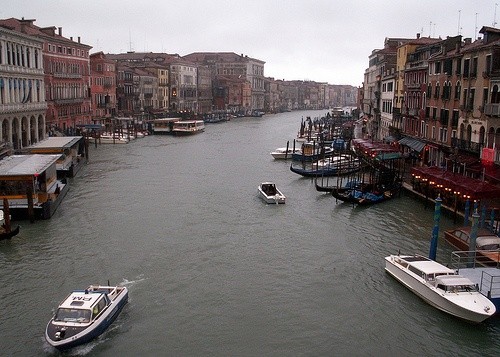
[0,153,69,219]
[202,111,263,124]
[257,181,285,204]
[85,129,149,144]
[289,107,398,206]
[45,279,129,350]
[270,147,301,159]
[172,120,204,136]
[16,134,86,178]
[385,249,496,323]
[154,117,178,135]
